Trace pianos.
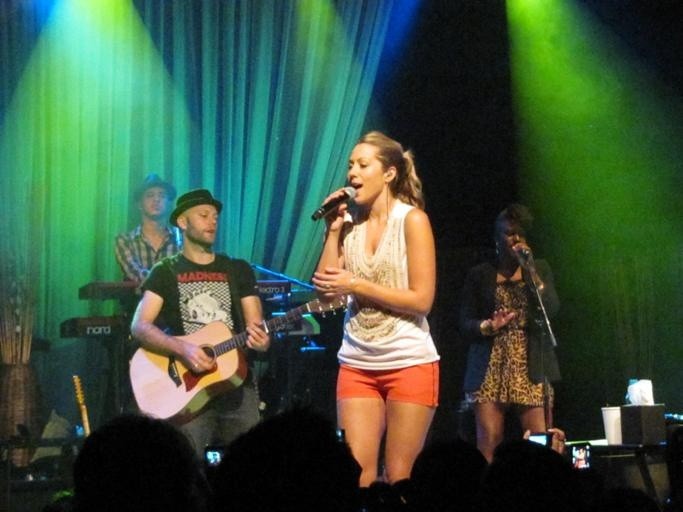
[61,279,322,338]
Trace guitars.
[72,375,93,489]
[129,293,348,427]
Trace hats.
[132,171,177,205]
[168,187,223,228]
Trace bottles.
[625,379,640,405]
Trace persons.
[441,203,562,462]
[128,187,271,458]
[42,406,676,511]
[114,174,184,293]
[307,129,441,488]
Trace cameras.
[563,444,591,470]
[528,434,549,449]
[205,446,222,466]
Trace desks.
[567,440,670,501]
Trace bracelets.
[479,319,488,336]
[534,282,544,292]
[350,273,359,293]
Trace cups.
[601,406,624,447]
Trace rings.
[325,282,331,289]
[192,363,198,368]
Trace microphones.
[311,187,356,223]
[519,247,531,257]
